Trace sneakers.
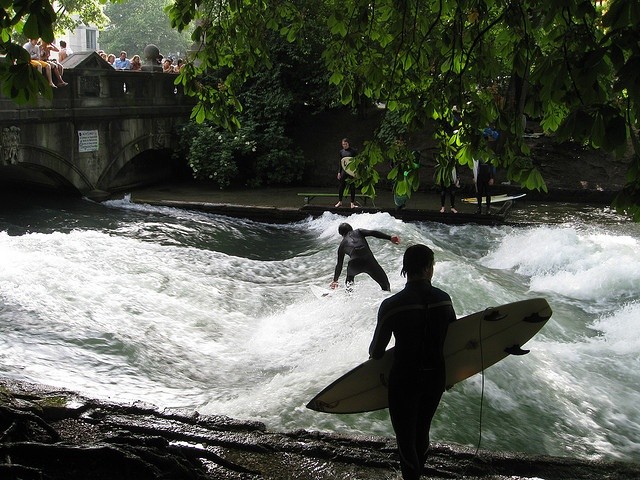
[486,211,491,215]
[475,209,481,214]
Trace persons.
[173,58,183,74]
[440,162,460,213]
[114,50,130,69]
[330,223,400,292]
[22,38,57,89]
[169,58,175,74]
[130,54,142,70]
[163,59,172,74]
[98,50,104,54]
[39,38,69,88]
[472,157,495,215]
[108,53,116,71]
[369,244,457,480]
[100,53,107,61]
[58,41,69,63]
[334,138,358,209]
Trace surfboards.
[471,193,526,204]
[393,149,422,206]
[305,298,553,414]
[460,194,508,201]
[452,166,460,188]
[341,157,379,179]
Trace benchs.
[297,192,377,208]
